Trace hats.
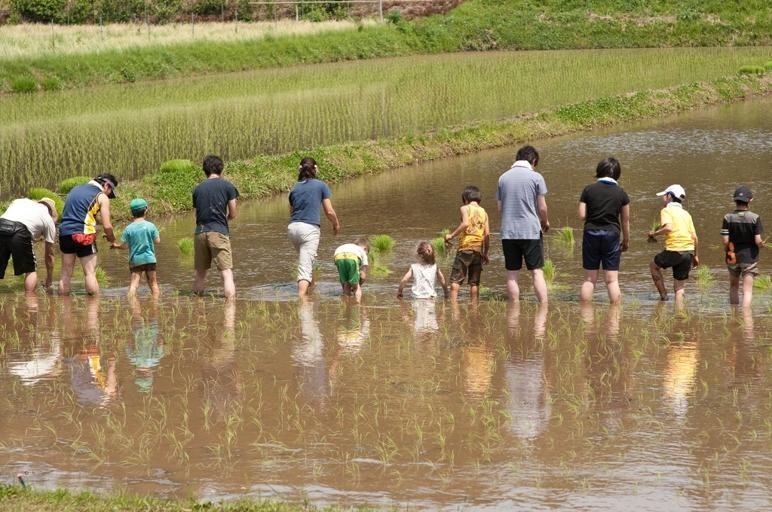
[97,176,116,199]
[130,199,147,210]
[734,187,752,202]
[656,184,686,201]
[32,197,58,224]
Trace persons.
[649,184,699,303]
[720,187,765,307]
[110,198,161,296]
[397,243,448,300]
[578,158,630,301]
[193,156,239,296]
[445,186,490,296]
[496,146,550,301]
[58,173,117,295]
[0,197,58,291]
[334,239,369,296]
[287,158,340,296]
